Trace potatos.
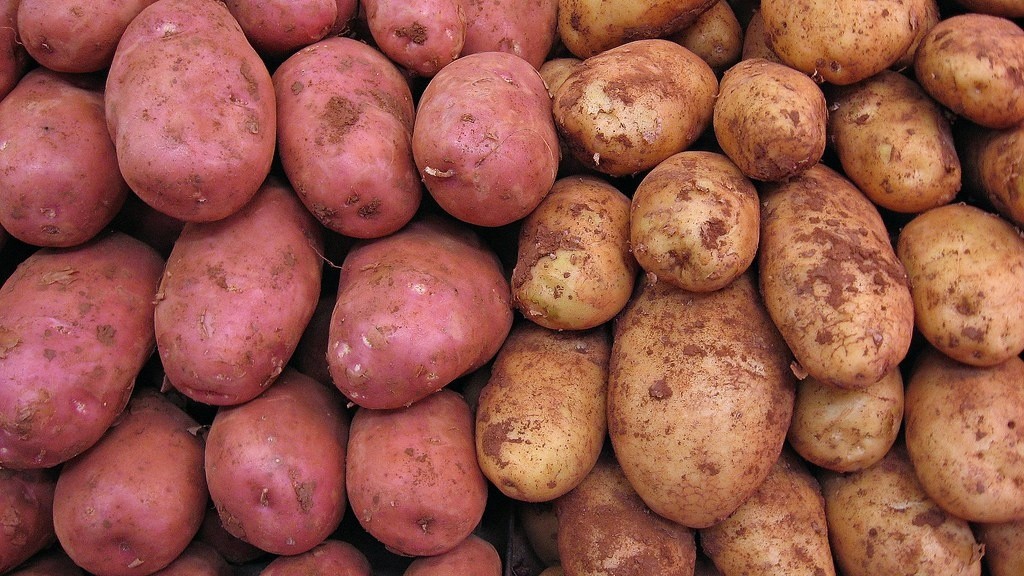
[0,0,1024,576]
